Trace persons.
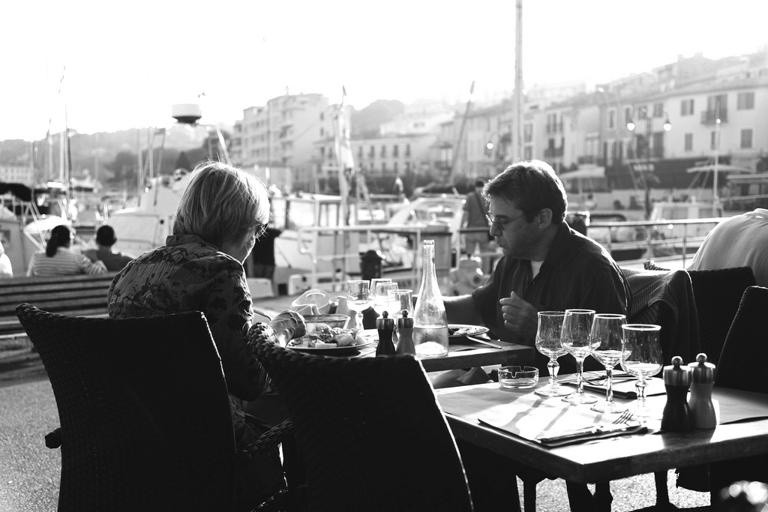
[79,225,135,273]
[611,186,677,210]
[686,207,768,288]
[265,173,404,202]
[106,160,308,494]
[459,179,492,275]
[27,224,109,352]
[411,159,635,390]
[0,241,13,278]
[583,193,598,222]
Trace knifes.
[541,420,640,444]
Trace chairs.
[247,323,521,512]
[15,302,293,512]
[620,264,768,512]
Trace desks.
[359,329,535,372]
[434,369,768,512]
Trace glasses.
[486,212,523,226]
[255,223,268,239]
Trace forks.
[456,331,502,349]
[537,408,634,441]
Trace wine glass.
[560,309,601,404]
[589,313,633,413]
[347,279,371,332]
[620,323,664,417]
[535,311,574,397]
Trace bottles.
[343,309,359,331]
[412,240,449,360]
[687,353,717,431]
[661,356,696,434]
[375,311,395,357]
[396,310,416,357]
[336,296,348,314]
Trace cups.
[370,278,415,325]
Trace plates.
[285,333,372,352]
[448,324,490,339]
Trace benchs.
[0,272,118,365]
